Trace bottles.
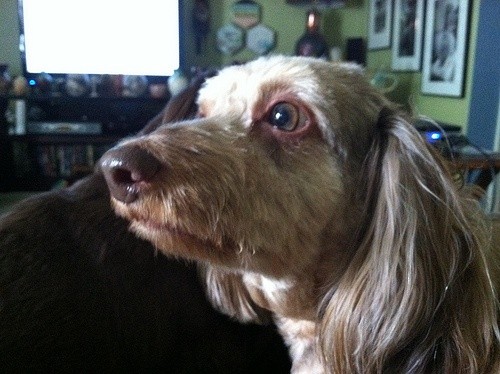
[0,65,14,122]
[295,10,328,59]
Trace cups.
[8,95,27,134]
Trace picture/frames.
[390,0,426,73]
[366,0,393,51]
[419,0,472,99]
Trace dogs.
[0,51,500,374]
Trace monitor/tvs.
[16,0,191,88]
